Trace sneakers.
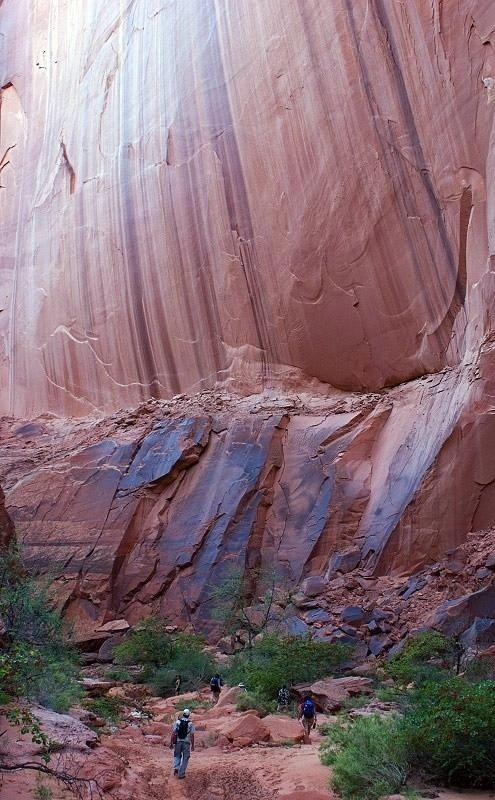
[173,770,186,779]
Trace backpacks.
[177,718,191,739]
[303,698,315,718]
[210,677,220,692]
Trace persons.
[175,674,181,696]
[210,673,248,703]
[169,708,194,779]
[277,684,317,737]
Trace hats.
[183,709,189,716]
[238,683,245,687]
